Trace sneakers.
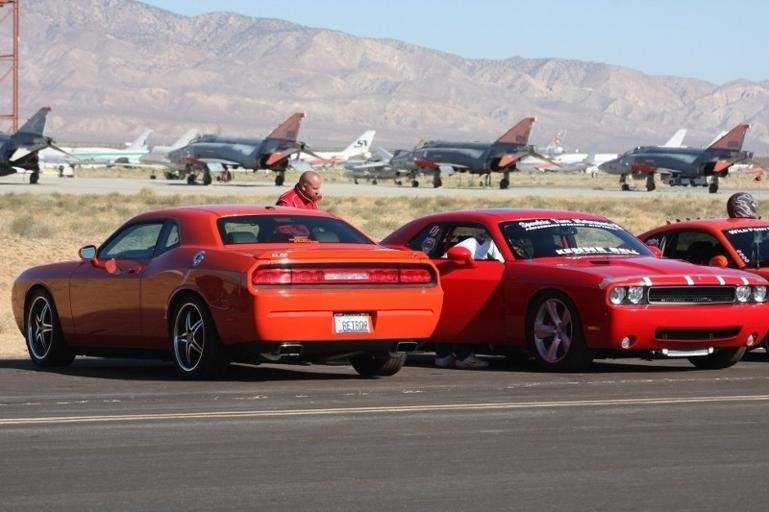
[435,353,455,367]
[456,355,490,370]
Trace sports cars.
[11,203,443,380]
[377,209,769,371]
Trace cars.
[615,217,769,356]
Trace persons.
[435,234,534,369]
[273,170,322,238]
